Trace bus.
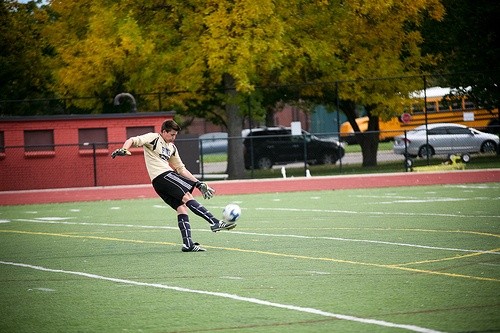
[336,87,500,144]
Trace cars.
[198,132,230,154]
[393,123,500,160]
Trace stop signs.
[400,113,411,124]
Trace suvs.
[241,125,347,170]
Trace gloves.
[111,148,131,159]
[196,180,215,199]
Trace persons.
[111,118,237,251]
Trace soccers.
[223,204,241,221]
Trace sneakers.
[182,243,206,252]
[210,220,237,232]
